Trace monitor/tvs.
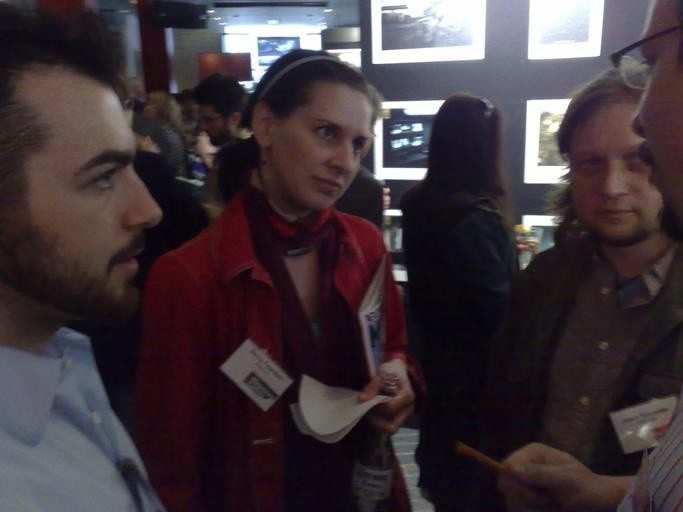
[256,36,301,67]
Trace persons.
[114,72,263,290]
[0,1,168,512]
[132,46,430,511]
[496,1,682,511]
[397,90,521,512]
[481,64,682,475]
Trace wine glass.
[516,227,543,264]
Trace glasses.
[608,22,681,91]
[468,95,497,140]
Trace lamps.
[320,0,361,54]
[203,0,229,26]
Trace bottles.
[349,374,396,510]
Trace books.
[355,254,388,384]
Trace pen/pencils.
[455,443,511,474]
